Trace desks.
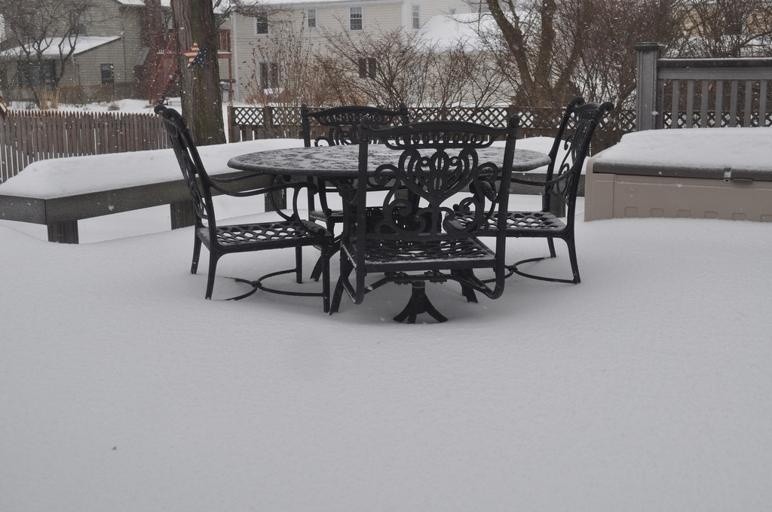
[226,143,551,315]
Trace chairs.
[443,101,615,298]
[155,103,335,315]
[301,104,411,281]
[330,113,521,325]
